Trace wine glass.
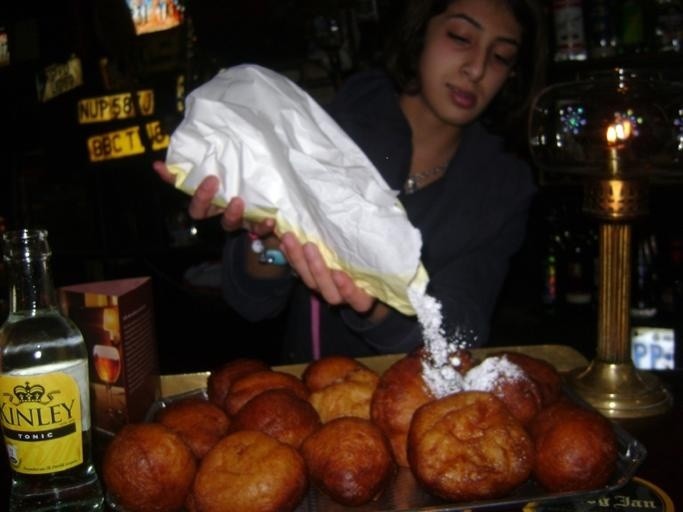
[91,343,121,415]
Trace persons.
[155,1,532,371]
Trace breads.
[99,345,619,512]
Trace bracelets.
[247,233,287,265]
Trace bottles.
[0,225,93,494]
[637,232,664,303]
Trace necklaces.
[403,162,446,196]
